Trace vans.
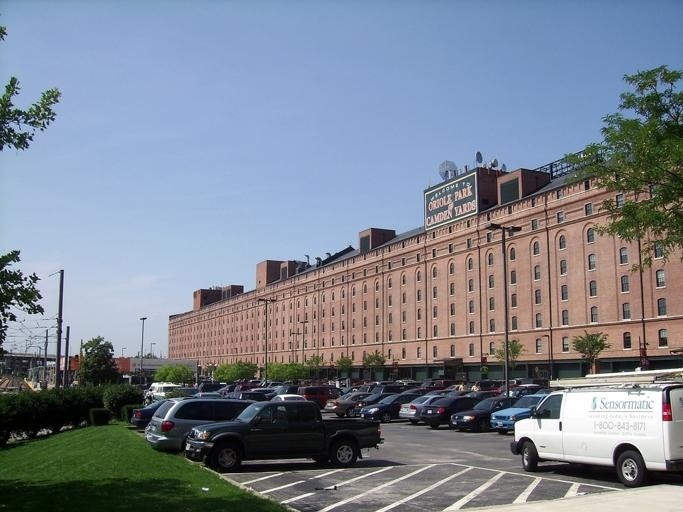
[510,367,683,487]
[144,396,269,461]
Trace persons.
[17,382,24,391]
[458,381,467,391]
[191,381,198,387]
[328,379,333,385]
[470,380,481,391]
[143,388,154,405]
[239,381,250,400]
[10,368,15,386]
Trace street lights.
[209,363,218,383]
[258,297,277,386]
[292,332,302,361]
[121,348,127,357]
[298,320,308,364]
[150,342,156,357]
[139,316,148,383]
[485,223,521,397]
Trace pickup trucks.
[184,401,384,473]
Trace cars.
[130,396,182,428]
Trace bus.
[123,375,132,385]
